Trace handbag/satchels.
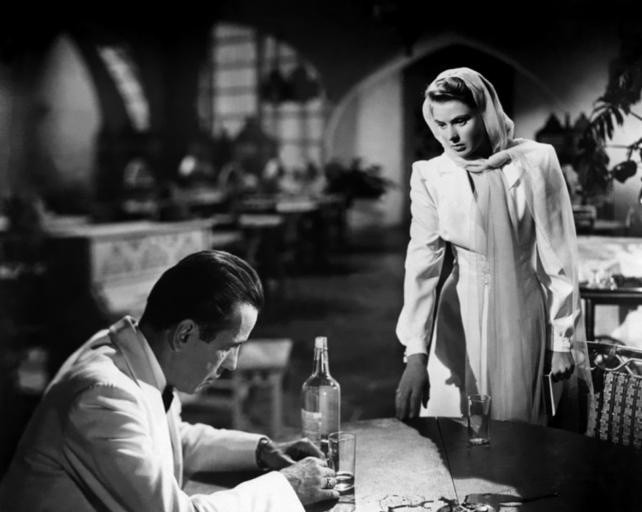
[584,352,641,449]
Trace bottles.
[299,336,340,473]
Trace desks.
[182,417,642,512]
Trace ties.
[163,385,174,413]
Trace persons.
[0,249,342,512]
[393,67,598,438]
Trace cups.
[466,392,492,446]
[326,430,356,495]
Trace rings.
[326,476,332,488]
